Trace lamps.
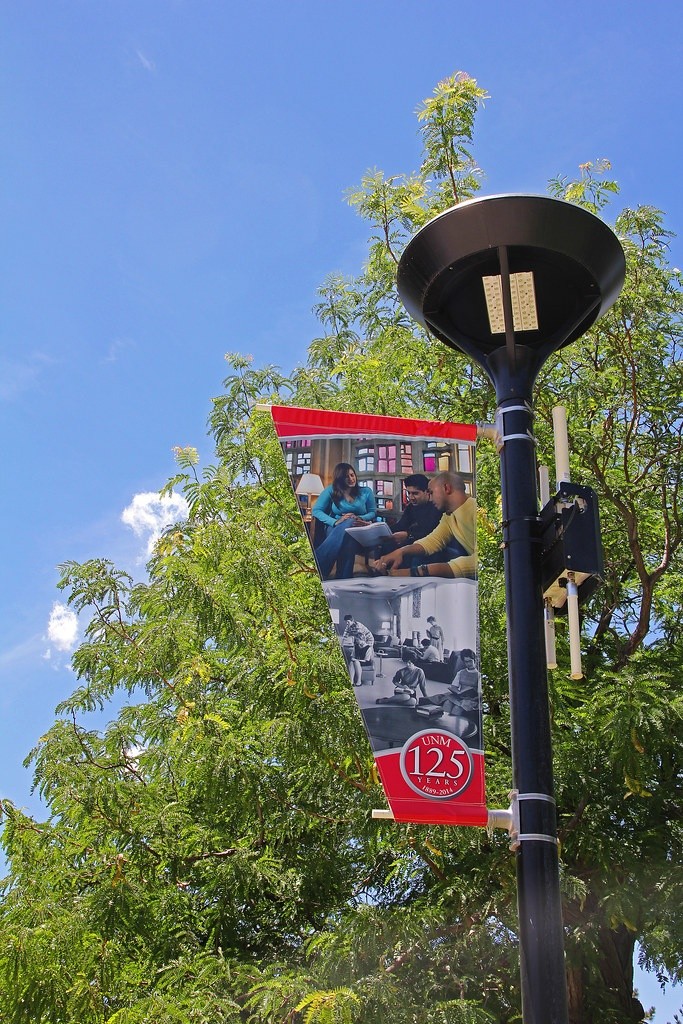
[296,474,324,523]
[382,622,390,631]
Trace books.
[345,522,393,548]
[447,686,478,700]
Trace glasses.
[463,658,475,663]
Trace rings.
[382,563,387,566]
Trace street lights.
[394,189,630,1024]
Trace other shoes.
[376,697,388,704]
[356,682,362,686]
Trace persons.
[415,640,440,662]
[373,472,478,579]
[311,463,377,581]
[376,651,427,706]
[443,649,480,716]
[340,615,374,649]
[349,632,372,686]
[426,616,444,662]
[379,474,443,549]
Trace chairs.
[347,648,376,686]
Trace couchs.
[419,650,462,685]
[375,634,399,658]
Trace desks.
[378,652,388,678]
[362,708,479,749]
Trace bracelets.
[410,564,429,577]
